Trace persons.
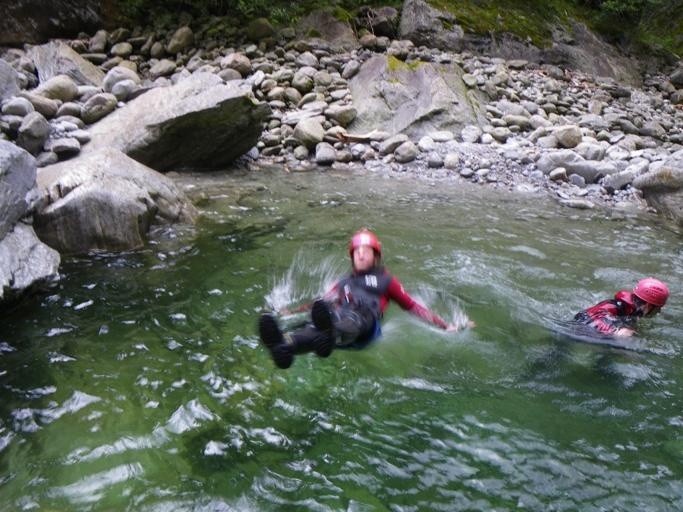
[258,228,476,369]
[574,277,670,337]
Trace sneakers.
[258,314,295,369]
[311,300,335,357]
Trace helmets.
[633,278,669,306]
[348,231,380,248]
